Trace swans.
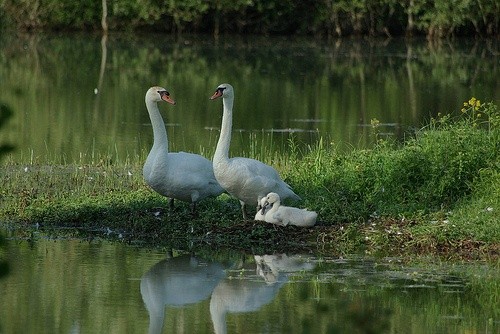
[253,254,316,284]
[70,247,227,334]
[210,249,286,334]
[263,192,318,227]
[254,197,307,222]
[210,83,302,226]
[143,86,230,221]
